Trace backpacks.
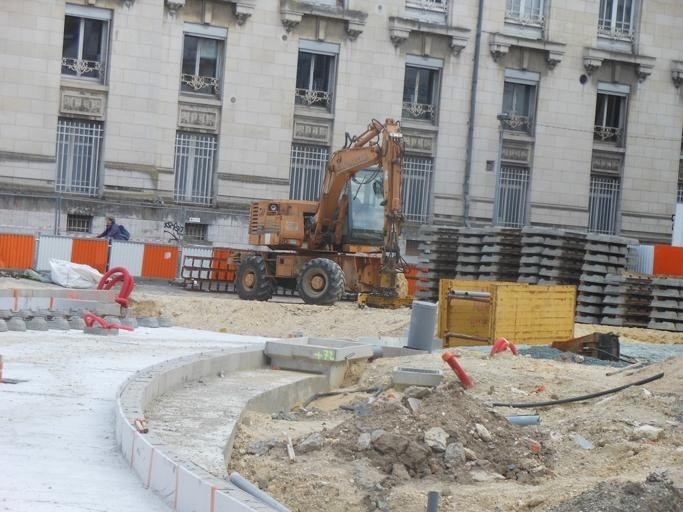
[119,225,130,240]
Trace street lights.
[489,112,514,225]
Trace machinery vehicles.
[224,117,413,313]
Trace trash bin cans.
[406,299,437,351]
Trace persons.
[96,215,123,240]
